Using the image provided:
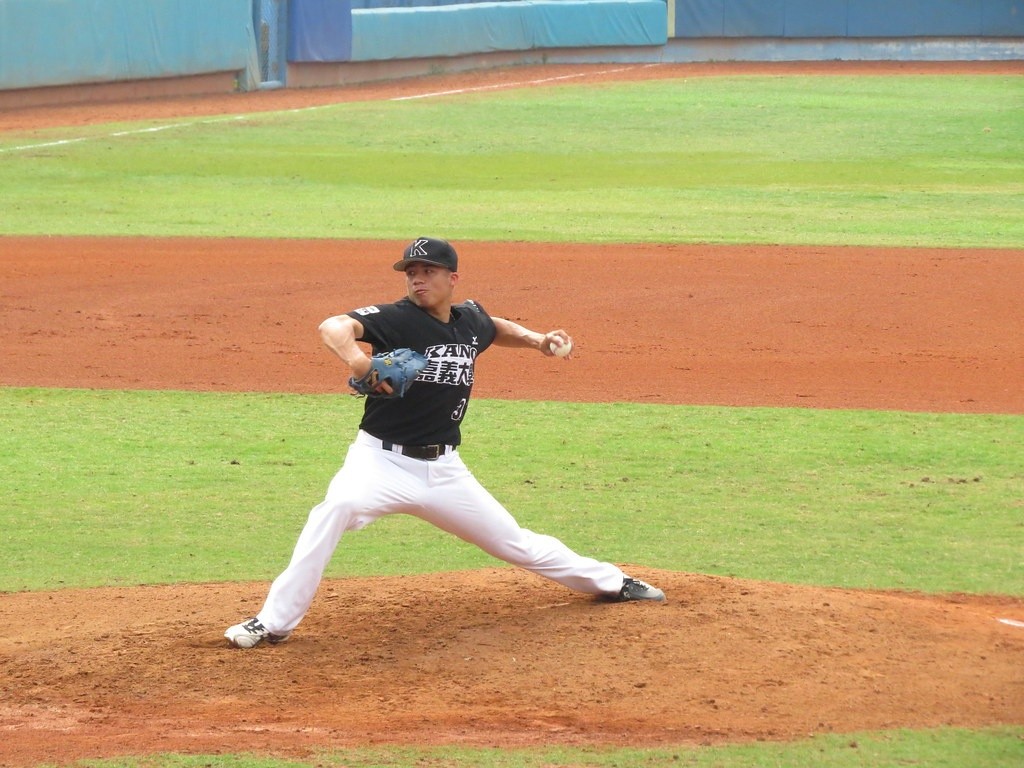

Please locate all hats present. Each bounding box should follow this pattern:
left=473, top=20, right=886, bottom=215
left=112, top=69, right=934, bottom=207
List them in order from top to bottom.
left=391, top=236, right=458, bottom=273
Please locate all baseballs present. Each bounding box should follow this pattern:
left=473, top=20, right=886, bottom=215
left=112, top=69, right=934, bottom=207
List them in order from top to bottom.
left=550, top=335, right=572, bottom=357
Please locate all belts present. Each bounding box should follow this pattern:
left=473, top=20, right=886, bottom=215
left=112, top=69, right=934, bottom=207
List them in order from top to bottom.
left=381, top=440, right=457, bottom=461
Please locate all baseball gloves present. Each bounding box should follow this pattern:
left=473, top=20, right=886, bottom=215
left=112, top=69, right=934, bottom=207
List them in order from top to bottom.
left=348, top=349, right=429, bottom=400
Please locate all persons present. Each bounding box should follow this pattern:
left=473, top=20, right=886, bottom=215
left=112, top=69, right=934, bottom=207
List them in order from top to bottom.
left=220, top=235, right=667, bottom=651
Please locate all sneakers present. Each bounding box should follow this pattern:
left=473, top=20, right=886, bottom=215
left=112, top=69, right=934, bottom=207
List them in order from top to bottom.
left=616, top=574, right=667, bottom=602
left=224, top=617, right=290, bottom=649
